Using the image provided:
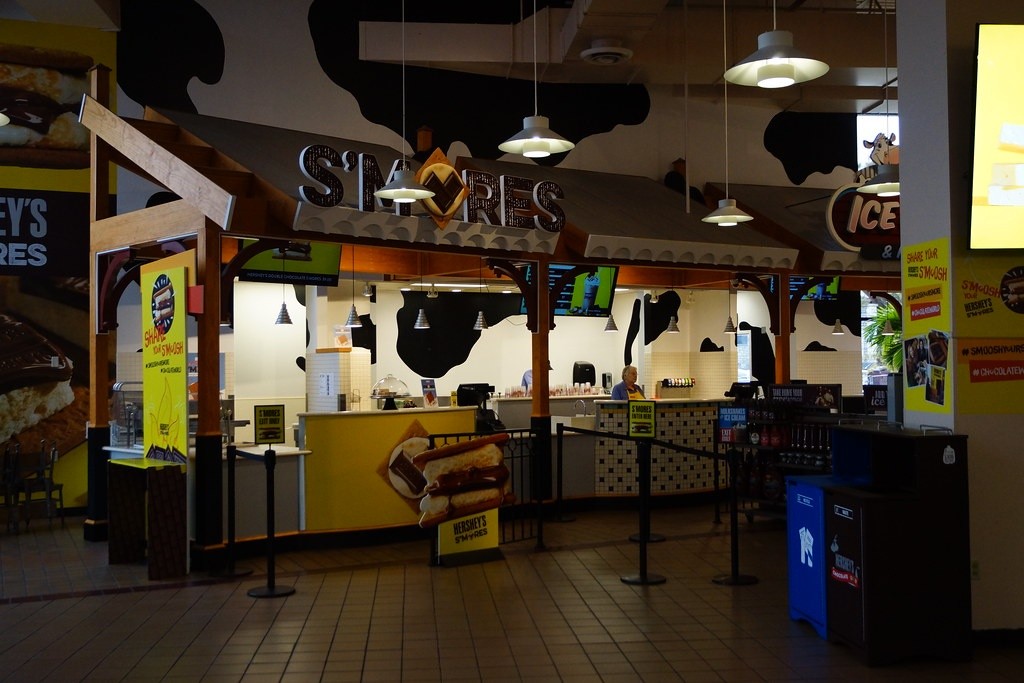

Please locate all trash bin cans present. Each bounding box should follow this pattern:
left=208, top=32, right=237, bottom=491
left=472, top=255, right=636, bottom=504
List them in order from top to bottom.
left=784, top=474, right=873, bottom=641
left=823, top=484, right=922, bottom=666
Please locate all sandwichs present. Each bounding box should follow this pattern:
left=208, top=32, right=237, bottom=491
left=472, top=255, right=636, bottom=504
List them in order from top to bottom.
left=0, top=41, right=95, bottom=170
left=0, top=309, right=75, bottom=444
left=412, top=433, right=517, bottom=528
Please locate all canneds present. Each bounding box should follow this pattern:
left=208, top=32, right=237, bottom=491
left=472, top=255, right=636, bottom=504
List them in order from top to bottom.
left=668, top=377, right=695, bottom=386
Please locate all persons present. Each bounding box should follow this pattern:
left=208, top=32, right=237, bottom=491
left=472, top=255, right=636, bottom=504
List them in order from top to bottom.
left=906, top=338, right=928, bottom=386
left=610, top=365, right=646, bottom=400
left=522, top=370, right=532, bottom=389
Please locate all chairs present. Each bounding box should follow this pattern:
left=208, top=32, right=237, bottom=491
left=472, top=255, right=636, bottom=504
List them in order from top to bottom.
left=0, top=438, right=65, bottom=535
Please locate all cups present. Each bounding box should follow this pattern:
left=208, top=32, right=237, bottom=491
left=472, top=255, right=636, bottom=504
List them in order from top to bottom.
left=583, top=275, right=600, bottom=311
left=505, top=382, right=600, bottom=399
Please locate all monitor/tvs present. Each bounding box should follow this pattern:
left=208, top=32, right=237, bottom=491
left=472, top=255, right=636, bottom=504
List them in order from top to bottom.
left=967, top=22, right=1024, bottom=255
left=236, top=239, right=342, bottom=289
left=520, top=264, right=619, bottom=318
left=771, top=275, right=841, bottom=301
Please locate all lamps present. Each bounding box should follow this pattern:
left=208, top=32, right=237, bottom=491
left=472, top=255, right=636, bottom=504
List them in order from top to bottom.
left=372, top=0, right=435, bottom=203
left=498, top=0, right=575, bottom=158
left=700, top=0, right=754, bottom=227
left=724, top=0, right=829, bottom=88
left=857, top=0, right=900, bottom=197
left=344, top=245, right=363, bottom=328
left=275, top=252, right=293, bottom=324
left=414, top=252, right=429, bottom=329
left=473, top=257, right=488, bottom=330
left=605, top=267, right=618, bottom=332
left=722, top=272, right=736, bottom=335
left=361, top=281, right=373, bottom=297
left=426, top=283, right=438, bottom=298
left=648, top=288, right=659, bottom=304
left=881, top=291, right=895, bottom=336
left=666, top=316, right=680, bottom=333
left=832, top=319, right=845, bottom=336
